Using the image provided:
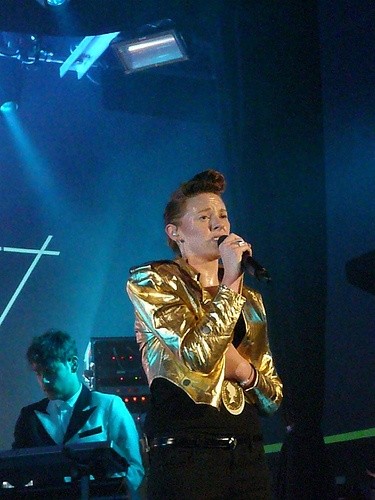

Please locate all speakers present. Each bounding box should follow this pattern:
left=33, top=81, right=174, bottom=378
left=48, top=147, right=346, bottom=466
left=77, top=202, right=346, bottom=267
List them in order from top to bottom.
left=83, top=337, right=149, bottom=414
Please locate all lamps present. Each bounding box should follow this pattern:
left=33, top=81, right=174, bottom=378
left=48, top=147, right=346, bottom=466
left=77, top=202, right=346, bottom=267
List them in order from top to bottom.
left=110, top=16, right=190, bottom=76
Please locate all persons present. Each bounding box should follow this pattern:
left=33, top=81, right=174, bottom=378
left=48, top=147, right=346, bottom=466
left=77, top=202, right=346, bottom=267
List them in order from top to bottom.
left=125, top=170, right=283, bottom=500
left=11, top=330, right=146, bottom=500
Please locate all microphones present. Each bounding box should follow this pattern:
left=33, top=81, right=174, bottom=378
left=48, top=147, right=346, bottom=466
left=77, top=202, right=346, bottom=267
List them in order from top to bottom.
left=217, top=235, right=273, bottom=284
